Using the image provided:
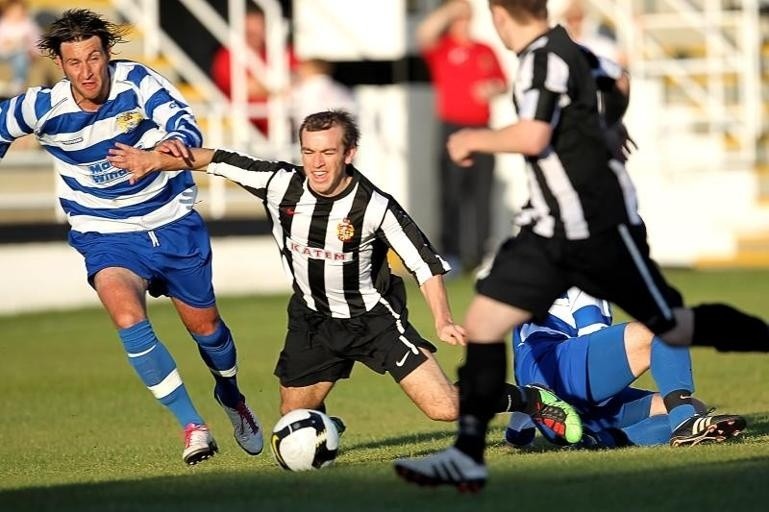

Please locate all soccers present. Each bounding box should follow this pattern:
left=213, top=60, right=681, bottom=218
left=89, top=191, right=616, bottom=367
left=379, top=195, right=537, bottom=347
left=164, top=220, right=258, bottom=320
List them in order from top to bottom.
left=270, top=409, right=340, bottom=472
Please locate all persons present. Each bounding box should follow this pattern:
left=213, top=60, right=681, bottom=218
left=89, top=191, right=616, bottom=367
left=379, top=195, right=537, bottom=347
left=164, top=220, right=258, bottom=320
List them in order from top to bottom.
left=388, top=0, right=768, bottom=495
left=556, top=0, right=629, bottom=118
left=0, top=1, right=43, bottom=92
left=415, top=1, right=508, bottom=274
left=205, top=5, right=324, bottom=142
left=0, top=6, right=265, bottom=467
left=104, top=109, right=585, bottom=451
left=505, top=283, right=747, bottom=450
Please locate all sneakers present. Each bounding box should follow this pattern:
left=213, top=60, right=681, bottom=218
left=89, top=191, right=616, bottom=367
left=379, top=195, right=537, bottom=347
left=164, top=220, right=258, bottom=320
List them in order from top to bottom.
left=526, top=383, right=584, bottom=444
left=214, top=385, right=263, bottom=455
left=669, top=408, right=747, bottom=448
left=182, top=422, right=218, bottom=466
left=573, top=426, right=604, bottom=451
left=393, top=447, right=488, bottom=494
